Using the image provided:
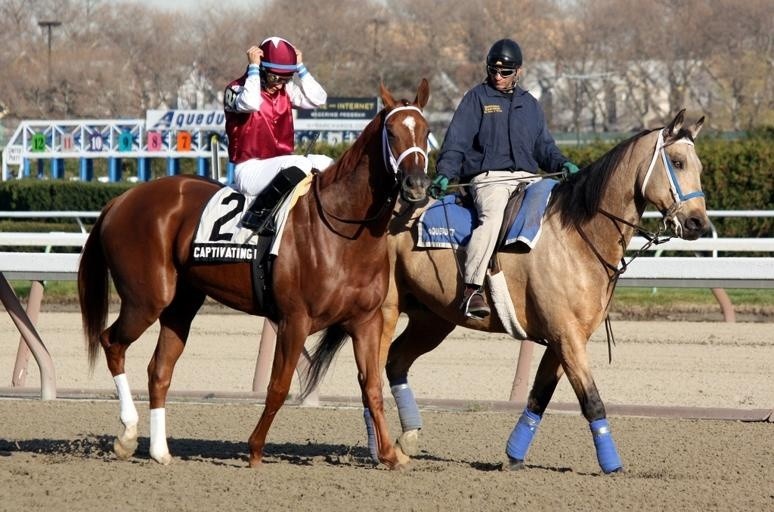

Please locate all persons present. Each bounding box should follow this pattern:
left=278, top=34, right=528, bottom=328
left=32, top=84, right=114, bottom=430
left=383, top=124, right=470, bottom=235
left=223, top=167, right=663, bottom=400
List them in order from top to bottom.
left=224, top=37, right=335, bottom=195
left=429, top=38, right=580, bottom=318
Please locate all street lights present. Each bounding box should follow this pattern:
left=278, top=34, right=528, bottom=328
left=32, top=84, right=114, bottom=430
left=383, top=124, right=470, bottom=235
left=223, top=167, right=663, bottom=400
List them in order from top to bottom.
left=361, top=16, right=393, bottom=102
left=36, top=19, right=66, bottom=119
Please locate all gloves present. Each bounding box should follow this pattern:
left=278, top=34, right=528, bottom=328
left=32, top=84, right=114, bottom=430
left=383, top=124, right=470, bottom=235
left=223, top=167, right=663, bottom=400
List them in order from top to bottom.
left=560, top=160, right=580, bottom=180
left=424, top=171, right=452, bottom=200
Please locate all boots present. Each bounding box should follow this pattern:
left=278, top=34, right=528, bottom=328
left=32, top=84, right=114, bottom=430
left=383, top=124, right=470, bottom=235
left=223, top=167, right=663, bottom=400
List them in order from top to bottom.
left=238, top=164, right=309, bottom=238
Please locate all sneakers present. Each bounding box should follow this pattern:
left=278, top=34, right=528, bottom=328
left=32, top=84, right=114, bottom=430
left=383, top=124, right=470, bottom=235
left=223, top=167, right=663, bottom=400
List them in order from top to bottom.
left=459, top=287, right=491, bottom=316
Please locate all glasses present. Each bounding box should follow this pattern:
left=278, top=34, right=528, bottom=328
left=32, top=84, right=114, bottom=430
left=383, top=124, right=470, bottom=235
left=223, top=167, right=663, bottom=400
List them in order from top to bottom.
left=487, top=67, right=516, bottom=78
left=268, top=74, right=292, bottom=84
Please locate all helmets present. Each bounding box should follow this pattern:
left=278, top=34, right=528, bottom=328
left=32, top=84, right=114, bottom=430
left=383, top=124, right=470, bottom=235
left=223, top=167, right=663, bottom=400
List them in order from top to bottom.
left=257, top=37, right=298, bottom=72
left=486, top=38, right=521, bottom=69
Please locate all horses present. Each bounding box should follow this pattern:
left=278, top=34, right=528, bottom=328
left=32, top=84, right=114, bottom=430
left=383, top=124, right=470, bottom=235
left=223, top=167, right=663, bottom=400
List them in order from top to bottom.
left=75, top=76, right=431, bottom=471
left=296, top=109, right=709, bottom=476
